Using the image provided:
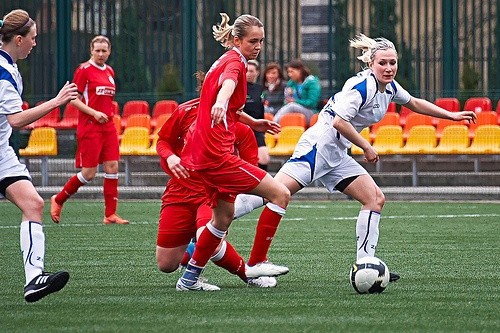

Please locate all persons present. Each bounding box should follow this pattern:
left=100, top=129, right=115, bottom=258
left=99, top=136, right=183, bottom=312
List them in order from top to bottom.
left=0, top=9, right=79, bottom=302
left=176, top=13, right=290, bottom=291
left=231, top=33, right=477, bottom=280
left=51, top=36, right=129, bottom=225
left=242, top=59, right=269, bottom=169
left=269, top=60, right=321, bottom=124
left=260, top=63, right=285, bottom=114
left=154, top=71, right=278, bottom=287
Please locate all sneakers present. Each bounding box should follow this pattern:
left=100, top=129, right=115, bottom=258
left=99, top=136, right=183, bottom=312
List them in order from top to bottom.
left=51, top=194, right=62, bottom=223
left=104, top=214, right=129, bottom=225
left=245, top=260, right=289, bottom=279
left=24, top=270, right=69, bottom=302
left=248, top=275, right=277, bottom=288
left=176, top=278, right=220, bottom=292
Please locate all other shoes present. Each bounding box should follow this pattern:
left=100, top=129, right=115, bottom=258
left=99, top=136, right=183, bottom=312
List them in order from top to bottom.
left=186, top=241, right=195, bottom=258
left=390, top=273, right=400, bottom=280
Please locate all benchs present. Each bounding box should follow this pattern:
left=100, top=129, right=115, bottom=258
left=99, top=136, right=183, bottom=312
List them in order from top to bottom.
left=269, top=97, right=500, bottom=186
left=20, top=101, right=178, bottom=187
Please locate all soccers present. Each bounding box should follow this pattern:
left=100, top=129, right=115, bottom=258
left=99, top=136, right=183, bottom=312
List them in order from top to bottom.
left=349, top=256, right=390, bottom=294
